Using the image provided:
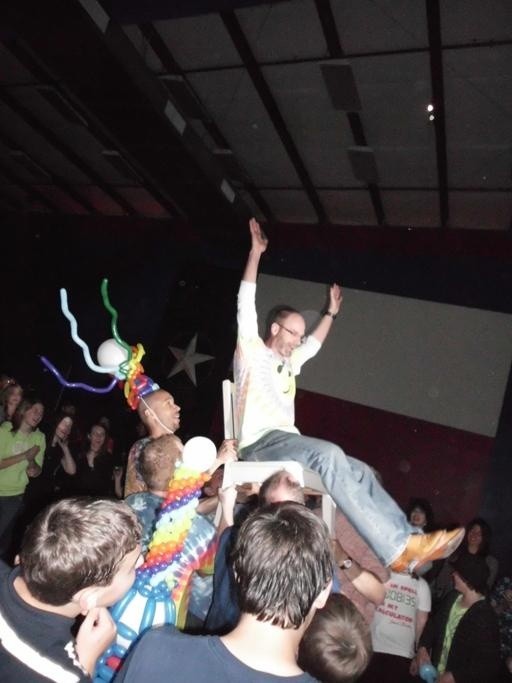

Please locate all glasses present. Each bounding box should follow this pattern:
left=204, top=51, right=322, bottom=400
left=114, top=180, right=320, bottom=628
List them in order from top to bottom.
left=280, top=323, right=307, bottom=343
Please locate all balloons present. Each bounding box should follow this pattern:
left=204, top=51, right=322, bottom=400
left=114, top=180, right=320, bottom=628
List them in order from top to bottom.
left=40, top=276, right=159, bottom=414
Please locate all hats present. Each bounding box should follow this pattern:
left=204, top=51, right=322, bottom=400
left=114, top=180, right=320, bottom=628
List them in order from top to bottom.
left=449, top=560, right=489, bottom=587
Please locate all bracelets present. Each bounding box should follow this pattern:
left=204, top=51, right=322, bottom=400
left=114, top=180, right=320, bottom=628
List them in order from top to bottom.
left=320, top=309, right=337, bottom=321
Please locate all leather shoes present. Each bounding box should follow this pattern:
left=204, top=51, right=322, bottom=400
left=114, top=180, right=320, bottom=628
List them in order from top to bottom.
left=413, top=526, right=466, bottom=577
left=390, top=528, right=445, bottom=572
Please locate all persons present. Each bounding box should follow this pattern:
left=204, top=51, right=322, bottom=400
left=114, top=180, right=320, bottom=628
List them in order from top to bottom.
left=233, top=217, right=468, bottom=574
left=1, top=376, right=512, bottom=683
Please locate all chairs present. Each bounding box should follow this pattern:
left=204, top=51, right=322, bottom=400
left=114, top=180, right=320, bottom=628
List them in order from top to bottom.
left=211, top=380, right=338, bottom=533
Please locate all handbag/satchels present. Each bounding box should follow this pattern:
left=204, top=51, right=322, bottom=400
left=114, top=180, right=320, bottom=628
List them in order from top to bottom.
left=39, top=469, right=76, bottom=501
